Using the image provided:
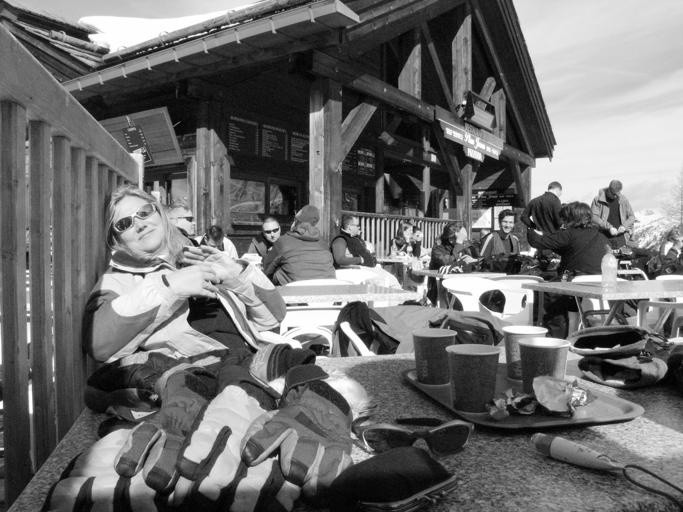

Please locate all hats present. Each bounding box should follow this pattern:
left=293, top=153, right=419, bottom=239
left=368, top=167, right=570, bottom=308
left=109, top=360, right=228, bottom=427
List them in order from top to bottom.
left=294, top=203, right=321, bottom=225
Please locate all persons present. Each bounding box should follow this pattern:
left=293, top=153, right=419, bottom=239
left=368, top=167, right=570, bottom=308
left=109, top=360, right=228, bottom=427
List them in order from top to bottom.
left=84, top=184, right=317, bottom=390
left=160, top=198, right=196, bottom=240
left=260, top=202, right=335, bottom=286
left=327, top=213, right=377, bottom=269
left=248, top=217, right=281, bottom=256
left=192, top=226, right=239, bottom=258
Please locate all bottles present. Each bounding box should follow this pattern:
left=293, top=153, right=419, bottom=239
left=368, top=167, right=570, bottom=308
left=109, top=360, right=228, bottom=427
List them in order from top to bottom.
left=599, top=247, right=619, bottom=287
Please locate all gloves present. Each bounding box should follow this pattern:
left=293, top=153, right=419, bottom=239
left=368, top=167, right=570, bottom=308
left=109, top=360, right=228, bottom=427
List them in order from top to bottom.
left=39, top=343, right=356, bottom=512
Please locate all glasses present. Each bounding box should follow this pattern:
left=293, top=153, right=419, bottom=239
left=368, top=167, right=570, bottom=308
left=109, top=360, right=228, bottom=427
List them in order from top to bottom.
left=109, top=201, right=159, bottom=233
left=345, top=223, right=360, bottom=228
left=263, top=227, right=280, bottom=234
left=350, top=406, right=473, bottom=455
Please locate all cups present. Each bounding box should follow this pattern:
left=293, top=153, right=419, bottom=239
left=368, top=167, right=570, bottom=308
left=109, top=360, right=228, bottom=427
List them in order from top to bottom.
left=502, top=325, right=549, bottom=382
left=412, top=328, right=457, bottom=388
left=519, top=336, right=571, bottom=398
left=444, top=343, right=499, bottom=415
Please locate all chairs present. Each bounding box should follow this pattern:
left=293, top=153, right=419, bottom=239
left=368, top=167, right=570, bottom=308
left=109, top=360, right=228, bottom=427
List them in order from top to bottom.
left=334, top=262, right=404, bottom=293
left=572, top=268, right=683, bottom=282
left=278, top=278, right=368, bottom=337
left=330, top=300, right=504, bottom=358
left=566, top=300, right=683, bottom=350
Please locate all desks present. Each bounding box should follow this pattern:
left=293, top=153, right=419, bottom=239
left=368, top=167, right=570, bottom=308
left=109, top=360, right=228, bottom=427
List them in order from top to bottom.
left=442, top=275, right=545, bottom=327
